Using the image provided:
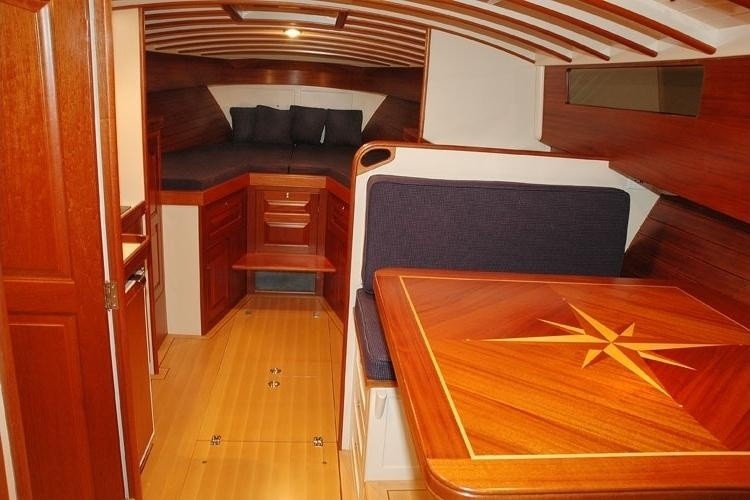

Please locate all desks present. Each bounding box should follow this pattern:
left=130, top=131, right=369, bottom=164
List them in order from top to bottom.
left=372, top=266, right=750, bottom=500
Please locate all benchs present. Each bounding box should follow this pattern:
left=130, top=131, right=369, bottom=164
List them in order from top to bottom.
left=348, top=174, right=630, bottom=482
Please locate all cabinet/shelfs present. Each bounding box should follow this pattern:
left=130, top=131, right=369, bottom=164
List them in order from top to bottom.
left=88, top=0, right=155, bottom=476
left=162, top=174, right=247, bottom=336
left=247, top=174, right=323, bottom=297
left=323, top=177, right=355, bottom=324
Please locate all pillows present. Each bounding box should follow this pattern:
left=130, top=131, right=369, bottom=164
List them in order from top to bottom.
left=256, top=104, right=296, bottom=148
left=290, top=104, right=326, bottom=149
left=230, top=106, right=255, bottom=147
left=326, top=109, right=363, bottom=148
